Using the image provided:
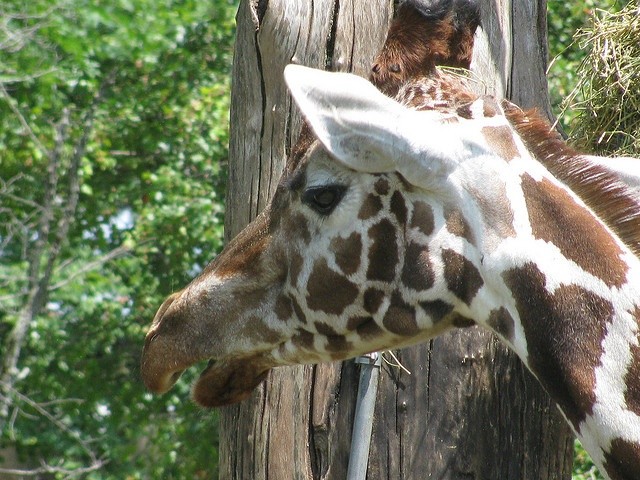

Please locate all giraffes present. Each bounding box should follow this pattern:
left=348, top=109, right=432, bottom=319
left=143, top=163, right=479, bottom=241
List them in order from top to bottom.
left=141, top=0, right=640, bottom=480
